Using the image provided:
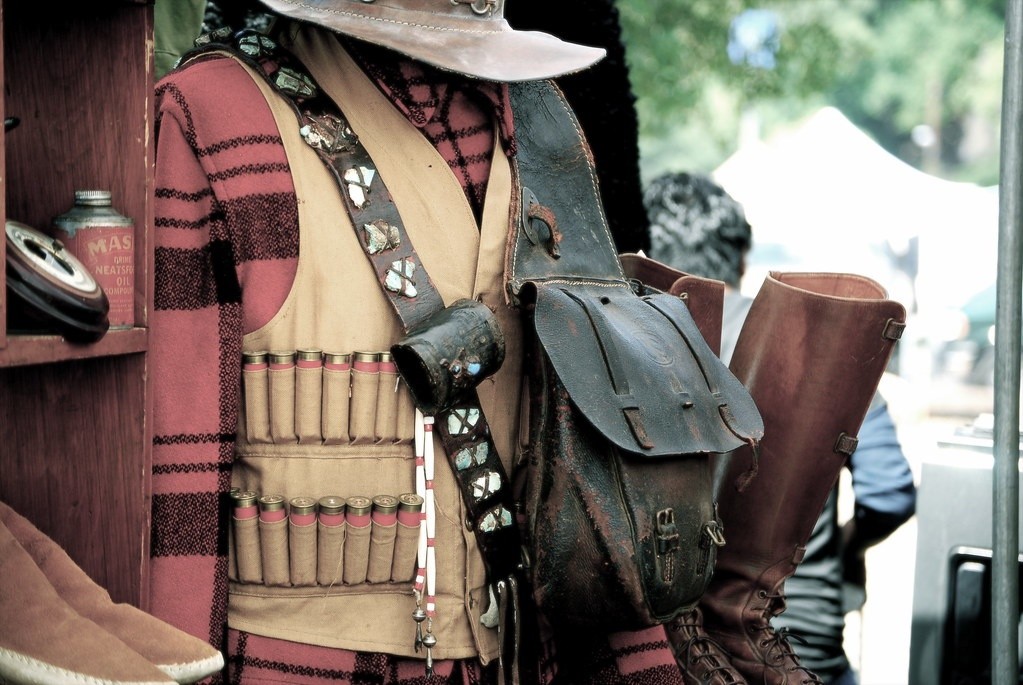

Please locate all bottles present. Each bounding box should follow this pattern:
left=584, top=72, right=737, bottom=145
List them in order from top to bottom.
left=54, top=190, right=135, bottom=329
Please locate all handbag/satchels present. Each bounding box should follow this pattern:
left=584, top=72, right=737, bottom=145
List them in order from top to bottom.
left=497, top=81, right=765, bottom=634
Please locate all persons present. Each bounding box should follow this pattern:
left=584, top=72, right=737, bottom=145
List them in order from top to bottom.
left=150, top=1, right=681, bottom=685
left=642, top=169, right=917, bottom=685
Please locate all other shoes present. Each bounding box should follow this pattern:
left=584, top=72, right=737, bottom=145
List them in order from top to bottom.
left=0, top=502, right=225, bottom=685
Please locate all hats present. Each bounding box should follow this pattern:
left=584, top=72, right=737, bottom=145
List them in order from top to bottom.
left=260, top=0, right=605, bottom=84
left=644, top=170, right=753, bottom=292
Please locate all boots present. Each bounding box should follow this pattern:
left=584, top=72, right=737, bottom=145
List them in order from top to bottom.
left=592, top=253, right=748, bottom=685
left=705, top=271, right=905, bottom=685
